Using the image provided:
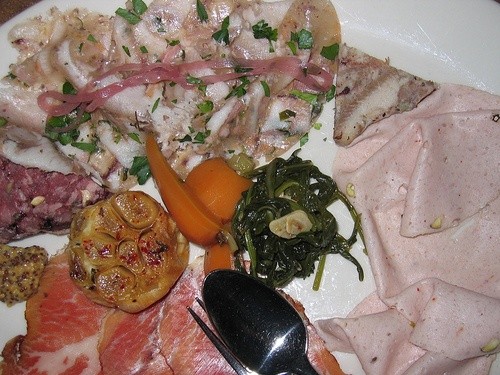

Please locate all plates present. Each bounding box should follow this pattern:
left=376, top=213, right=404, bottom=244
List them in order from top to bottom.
left=0, top=0, right=499, bottom=375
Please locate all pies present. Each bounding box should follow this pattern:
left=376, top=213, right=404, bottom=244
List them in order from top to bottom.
left=316, top=84, right=500, bottom=374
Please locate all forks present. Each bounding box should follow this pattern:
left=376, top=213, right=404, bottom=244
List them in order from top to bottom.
left=188, top=298, right=252, bottom=375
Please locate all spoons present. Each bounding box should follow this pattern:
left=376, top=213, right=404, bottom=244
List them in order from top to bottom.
left=202, top=269, right=321, bottom=375
